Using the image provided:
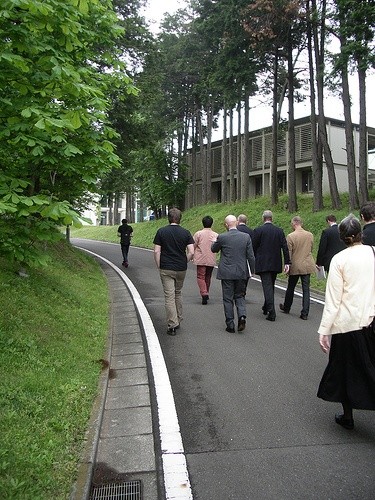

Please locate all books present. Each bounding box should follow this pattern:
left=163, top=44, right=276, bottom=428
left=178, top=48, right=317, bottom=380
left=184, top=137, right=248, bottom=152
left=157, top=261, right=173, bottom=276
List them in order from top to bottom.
left=317, top=266, right=326, bottom=279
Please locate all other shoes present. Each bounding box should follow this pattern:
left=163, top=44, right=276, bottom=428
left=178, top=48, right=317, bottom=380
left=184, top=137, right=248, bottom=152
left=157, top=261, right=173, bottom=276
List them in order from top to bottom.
left=122, top=261, right=128, bottom=267
left=202, top=295, right=209, bottom=304
left=280, top=303, right=289, bottom=313
left=262, top=306, right=267, bottom=315
left=300, top=315, right=307, bottom=319
left=237, top=317, right=246, bottom=331
left=266, top=317, right=275, bottom=321
left=167, top=322, right=180, bottom=336
left=226, top=328, right=235, bottom=333
left=335, top=414, right=354, bottom=429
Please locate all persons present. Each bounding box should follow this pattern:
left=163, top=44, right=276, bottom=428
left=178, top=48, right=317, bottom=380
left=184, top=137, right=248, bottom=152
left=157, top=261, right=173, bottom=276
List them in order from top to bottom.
left=316, top=206, right=375, bottom=430
left=153, top=208, right=195, bottom=335
left=251, top=210, right=316, bottom=321
left=211, top=214, right=256, bottom=333
left=191, top=216, right=220, bottom=305
left=117, top=219, right=134, bottom=267
